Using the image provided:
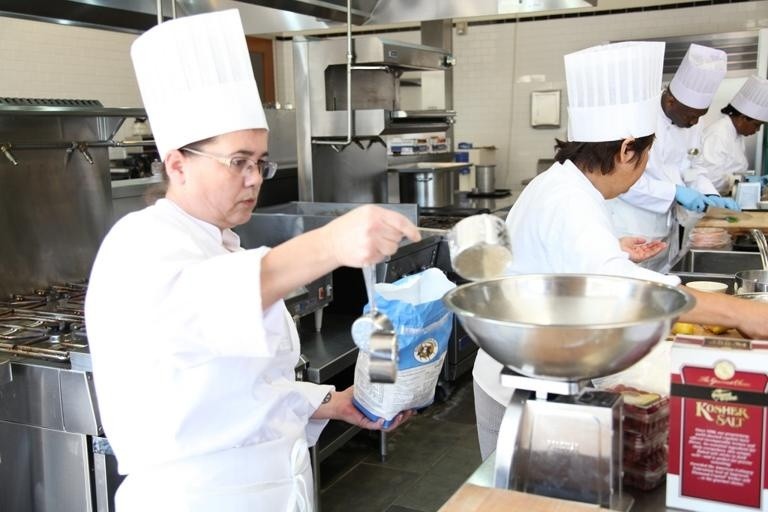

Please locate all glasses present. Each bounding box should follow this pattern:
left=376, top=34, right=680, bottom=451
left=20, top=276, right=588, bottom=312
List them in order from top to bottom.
left=180, top=145, right=278, bottom=182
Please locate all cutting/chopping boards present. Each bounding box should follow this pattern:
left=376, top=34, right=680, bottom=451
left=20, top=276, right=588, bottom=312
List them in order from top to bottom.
left=695, top=211, right=768, bottom=236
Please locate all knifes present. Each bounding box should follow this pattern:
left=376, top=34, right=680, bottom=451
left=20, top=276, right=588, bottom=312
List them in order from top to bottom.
left=676, top=200, right=752, bottom=220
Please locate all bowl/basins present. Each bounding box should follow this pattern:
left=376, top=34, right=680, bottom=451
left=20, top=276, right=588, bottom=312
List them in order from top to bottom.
left=445, top=273, right=696, bottom=381
left=686, top=281, right=729, bottom=294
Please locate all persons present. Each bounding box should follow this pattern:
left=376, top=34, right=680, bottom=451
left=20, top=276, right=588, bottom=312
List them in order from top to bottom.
left=81, top=6, right=422, bottom=511
left=470, top=38, right=768, bottom=463
left=608, top=43, right=743, bottom=270
left=701, top=76, right=768, bottom=189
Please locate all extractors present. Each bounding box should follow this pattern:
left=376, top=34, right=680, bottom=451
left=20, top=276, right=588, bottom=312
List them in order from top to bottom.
left=0, top=2, right=173, bottom=116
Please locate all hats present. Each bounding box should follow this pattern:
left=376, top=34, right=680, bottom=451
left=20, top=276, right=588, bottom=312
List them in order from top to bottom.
left=129, top=7, right=269, bottom=161
left=565, top=41, right=665, bottom=142
left=669, top=43, right=728, bottom=110
left=731, top=74, right=768, bottom=124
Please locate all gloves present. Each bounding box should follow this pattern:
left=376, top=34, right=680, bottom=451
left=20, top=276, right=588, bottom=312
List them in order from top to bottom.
left=745, top=176, right=768, bottom=188
left=676, top=186, right=716, bottom=214
left=708, top=195, right=742, bottom=214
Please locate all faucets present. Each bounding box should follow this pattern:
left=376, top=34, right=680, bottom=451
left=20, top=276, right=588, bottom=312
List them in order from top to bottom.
left=748, top=229, right=768, bottom=270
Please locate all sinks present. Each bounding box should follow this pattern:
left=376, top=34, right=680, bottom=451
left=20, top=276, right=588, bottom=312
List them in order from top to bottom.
left=668, top=246, right=766, bottom=275
left=675, top=279, right=742, bottom=299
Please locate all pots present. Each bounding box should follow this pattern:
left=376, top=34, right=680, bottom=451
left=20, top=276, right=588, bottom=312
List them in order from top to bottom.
left=413, top=171, right=454, bottom=208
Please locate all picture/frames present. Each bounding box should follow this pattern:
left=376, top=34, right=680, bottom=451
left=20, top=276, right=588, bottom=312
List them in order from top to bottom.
left=530, top=90, right=562, bottom=128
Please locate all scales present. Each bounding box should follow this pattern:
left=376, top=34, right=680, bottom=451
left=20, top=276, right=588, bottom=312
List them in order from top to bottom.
left=442, top=275, right=697, bottom=508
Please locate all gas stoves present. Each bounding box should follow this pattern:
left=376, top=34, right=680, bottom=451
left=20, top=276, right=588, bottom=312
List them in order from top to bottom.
left=0, top=278, right=93, bottom=372
